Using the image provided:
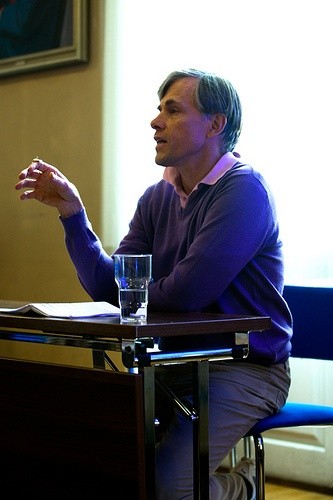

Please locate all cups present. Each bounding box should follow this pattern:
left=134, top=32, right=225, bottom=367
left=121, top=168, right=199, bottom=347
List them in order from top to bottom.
left=113, top=253, right=152, bottom=325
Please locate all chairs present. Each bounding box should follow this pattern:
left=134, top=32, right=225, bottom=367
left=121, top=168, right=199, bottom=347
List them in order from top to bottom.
left=244, top=286, right=333, bottom=500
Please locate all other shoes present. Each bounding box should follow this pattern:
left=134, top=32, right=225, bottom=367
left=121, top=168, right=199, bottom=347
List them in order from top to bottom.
left=234, top=457, right=256, bottom=500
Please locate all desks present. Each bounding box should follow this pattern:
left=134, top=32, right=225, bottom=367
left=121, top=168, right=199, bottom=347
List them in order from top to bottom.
left=0, top=299, right=270, bottom=500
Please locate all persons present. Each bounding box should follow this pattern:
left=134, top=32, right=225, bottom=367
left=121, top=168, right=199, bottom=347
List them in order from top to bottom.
left=15, top=68, right=293, bottom=500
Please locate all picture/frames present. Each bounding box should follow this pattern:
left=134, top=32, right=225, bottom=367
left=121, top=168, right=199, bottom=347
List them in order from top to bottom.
left=0, top=0, right=90, bottom=79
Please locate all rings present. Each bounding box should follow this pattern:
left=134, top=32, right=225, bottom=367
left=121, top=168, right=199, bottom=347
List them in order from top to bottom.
left=32, top=156, right=42, bottom=163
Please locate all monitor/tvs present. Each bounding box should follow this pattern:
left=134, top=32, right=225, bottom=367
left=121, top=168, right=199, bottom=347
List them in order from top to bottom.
left=0, top=0, right=89, bottom=76
